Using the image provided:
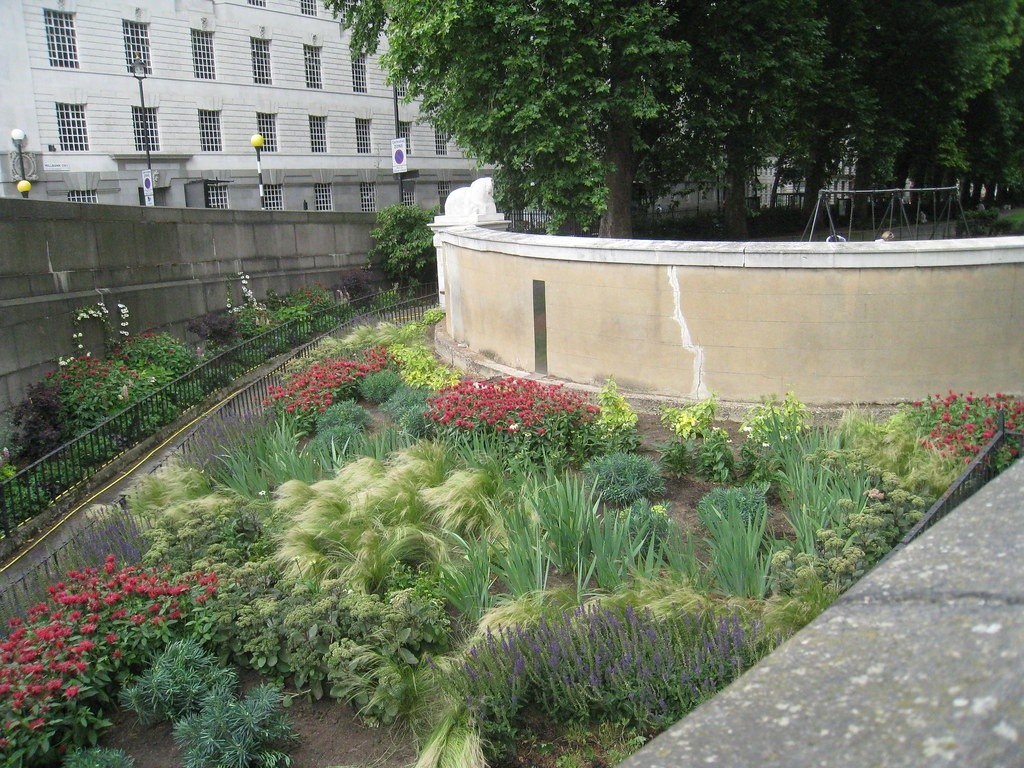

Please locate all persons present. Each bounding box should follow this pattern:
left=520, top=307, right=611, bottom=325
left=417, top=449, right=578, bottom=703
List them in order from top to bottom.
left=976, top=200, right=985, bottom=210
left=656, top=204, right=662, bottom=217
left=875, top=231, right=894, bottom=241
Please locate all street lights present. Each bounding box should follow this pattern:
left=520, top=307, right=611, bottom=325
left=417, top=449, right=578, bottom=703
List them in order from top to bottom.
left=130, top=56, right=152, bottom=171
left=11, top=128, right=26, bottom=181
left=250, top=135, right=267, bottom=210
